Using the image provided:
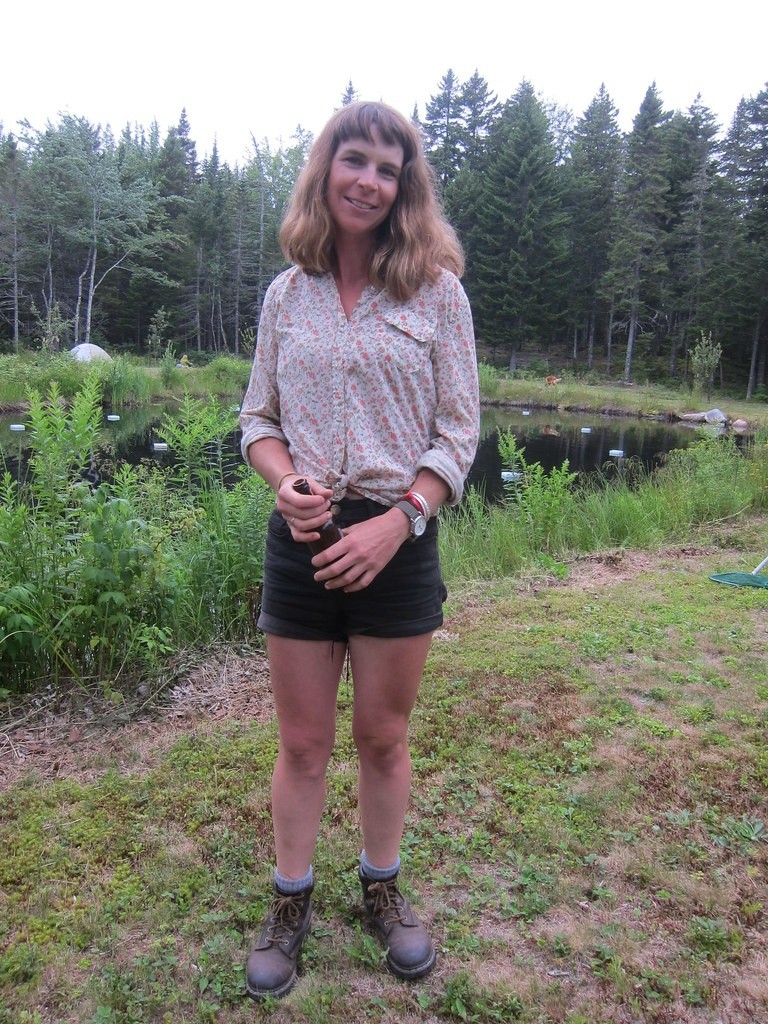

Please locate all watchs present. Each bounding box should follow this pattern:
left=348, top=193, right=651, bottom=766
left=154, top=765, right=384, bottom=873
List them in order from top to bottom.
left=394, top=500, right=426, bottom=539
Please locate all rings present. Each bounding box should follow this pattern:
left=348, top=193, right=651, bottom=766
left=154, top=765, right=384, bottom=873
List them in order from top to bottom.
left=289, top=517, right=295, bottom=525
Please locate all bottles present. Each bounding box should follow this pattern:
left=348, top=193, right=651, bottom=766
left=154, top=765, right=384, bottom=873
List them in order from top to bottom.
left=292, top=478, right=346, bottom=591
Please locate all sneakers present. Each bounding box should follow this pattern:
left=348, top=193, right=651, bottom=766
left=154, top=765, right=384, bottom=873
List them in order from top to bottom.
left=358, top=867, right=436, bottom=978
left=245, top=883, right=313, bottom=1001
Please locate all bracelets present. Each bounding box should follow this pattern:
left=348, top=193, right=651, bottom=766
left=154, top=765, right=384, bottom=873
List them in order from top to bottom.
left=278, top=472, right=300, bottom=490
left=398, top=492, right=430, bottom=522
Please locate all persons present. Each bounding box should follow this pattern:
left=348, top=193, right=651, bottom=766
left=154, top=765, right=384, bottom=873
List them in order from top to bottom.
left=237, top=100, right=479, bottom=1003
